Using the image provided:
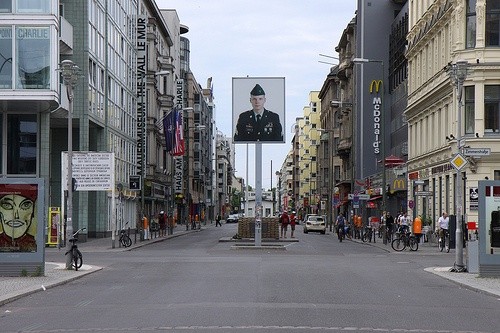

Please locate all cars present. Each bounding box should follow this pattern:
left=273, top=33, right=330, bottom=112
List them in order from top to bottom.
left=226, top=213, right=245, bottom=223
left=268, top=214, right=299, bottom=224
left=304, top=214, right=326, bottom=235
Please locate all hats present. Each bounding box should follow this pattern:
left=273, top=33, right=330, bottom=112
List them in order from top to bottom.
left=250, top=84, right=265, bottom=96
left=284, top=210, right=287, bottom=212
left=292, top=211, right=296, bottom=213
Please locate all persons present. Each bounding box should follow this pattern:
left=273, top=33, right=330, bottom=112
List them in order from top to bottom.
left=234, top=83, right=283, bottom=141
left=142, top=211, right=168, bottom=240
left=280, top=211, right=289, bottom=238
left=289, top=211, right=297, bottom=237
left=335, top=211, right=450, bottom=250
left=216, top=213, right=222, bottom=227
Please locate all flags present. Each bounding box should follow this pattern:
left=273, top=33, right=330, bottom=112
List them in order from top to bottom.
left=164, top=106, right=184, bottom=156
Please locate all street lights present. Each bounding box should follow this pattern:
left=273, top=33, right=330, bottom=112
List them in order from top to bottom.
left=352, top=58, right=388, bottom=243
left=55, top=60, right=82, bottom=270
left=449, top=61, right=469, bottom=273
left=140, top=70, right=169, bottom=242
left=169, top=108, right=194, bottom=234
left=185, top=125, right=206, bottom=231
left=317, top=129, right=334, bottom=231
left=210, top=158, right=222, bottom=223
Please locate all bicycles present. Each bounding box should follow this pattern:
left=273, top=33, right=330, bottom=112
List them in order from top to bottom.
left=337, top=222, right=372, bottom=242
left=118, top=223, right=132, bottom=247
left=379, top=223, right=418, bottom=252
left=65, top=227, right=87, bottom=271
left=439, top=228, right=450, bottom=253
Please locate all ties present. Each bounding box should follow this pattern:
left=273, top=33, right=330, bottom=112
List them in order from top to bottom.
left=256, top=114, right=261, bottom=122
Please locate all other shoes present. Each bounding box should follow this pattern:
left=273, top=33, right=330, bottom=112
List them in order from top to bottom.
left=291, top=236, right=295, bottom=238
left=280, top=237, right=282, bottom=238
left=284, top=237, right=287, bottom=238
